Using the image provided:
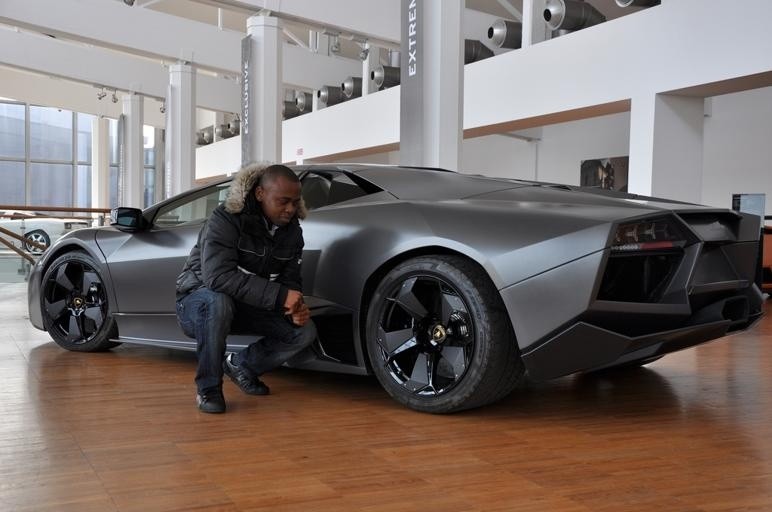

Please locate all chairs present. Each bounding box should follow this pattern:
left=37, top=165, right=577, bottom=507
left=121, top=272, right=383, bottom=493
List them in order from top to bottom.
left=301, top=176, right=330, bottom=207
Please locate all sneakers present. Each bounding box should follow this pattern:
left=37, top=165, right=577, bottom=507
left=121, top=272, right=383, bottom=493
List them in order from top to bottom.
left=221, top=352, right=270, bottom=396
left=194, top=390, right=227, bottom=414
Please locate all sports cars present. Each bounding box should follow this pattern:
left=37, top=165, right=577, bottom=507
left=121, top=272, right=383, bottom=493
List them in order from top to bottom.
left=22, top=157, right=767, bottom=417
left=0, top=208, right=90, bottom=259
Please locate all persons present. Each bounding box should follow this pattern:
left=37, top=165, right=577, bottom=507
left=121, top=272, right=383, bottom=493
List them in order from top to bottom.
left=174, top=162, right=317, bottom=414
